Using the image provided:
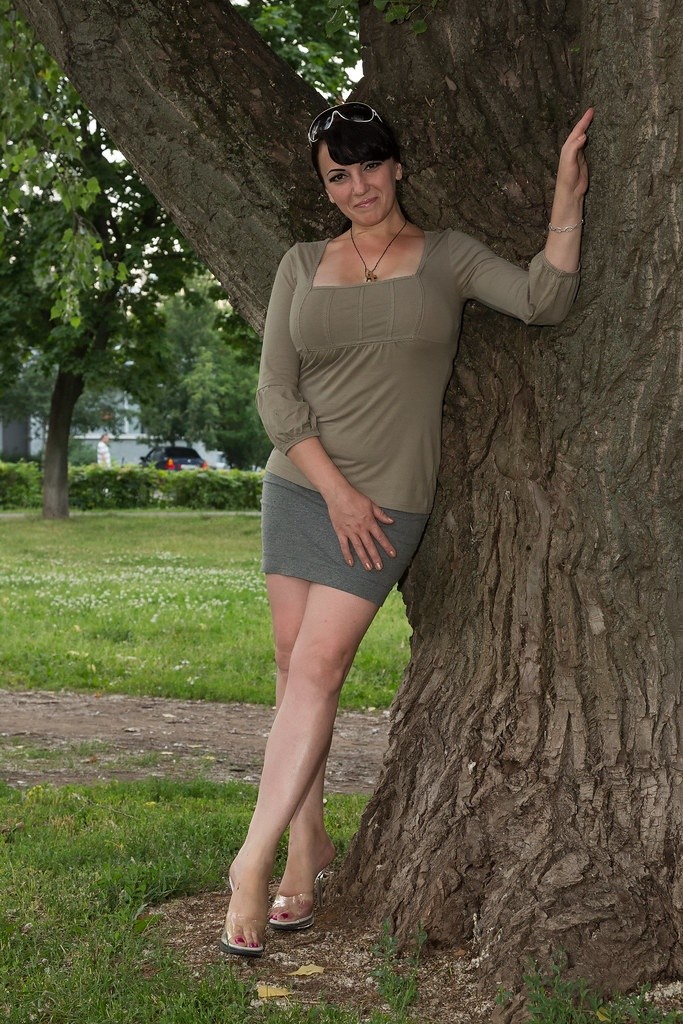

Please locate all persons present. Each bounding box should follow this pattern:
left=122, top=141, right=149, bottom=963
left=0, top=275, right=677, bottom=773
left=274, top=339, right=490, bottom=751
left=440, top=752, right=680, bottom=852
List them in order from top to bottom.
left=218, top=102, right=597, bottom=958
left=96, top=434, right=113, bottom=467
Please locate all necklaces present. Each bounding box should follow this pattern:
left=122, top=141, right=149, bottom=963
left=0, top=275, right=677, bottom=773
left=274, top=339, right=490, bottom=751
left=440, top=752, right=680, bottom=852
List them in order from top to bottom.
left=349, top=220, right=409, bottom=282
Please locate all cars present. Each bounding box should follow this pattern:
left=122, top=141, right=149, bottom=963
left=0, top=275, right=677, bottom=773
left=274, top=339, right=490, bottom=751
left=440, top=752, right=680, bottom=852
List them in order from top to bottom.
left=139, top=445, right=206, bottom=471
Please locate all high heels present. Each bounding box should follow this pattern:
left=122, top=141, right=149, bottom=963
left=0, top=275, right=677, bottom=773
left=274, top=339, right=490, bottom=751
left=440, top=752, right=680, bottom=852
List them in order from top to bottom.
left=268, top=867, right=328, bottom=932
left=221, top=876, right=265, bottom=954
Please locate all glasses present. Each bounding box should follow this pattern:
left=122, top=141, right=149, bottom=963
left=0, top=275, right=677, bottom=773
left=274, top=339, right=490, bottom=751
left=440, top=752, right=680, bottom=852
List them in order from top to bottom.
left=307, top=102, right=381, bottom=150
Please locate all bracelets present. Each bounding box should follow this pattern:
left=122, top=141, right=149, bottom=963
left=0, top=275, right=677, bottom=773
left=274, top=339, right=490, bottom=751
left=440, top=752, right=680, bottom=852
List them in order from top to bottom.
left=549, top=219, right=584, bottom=233
